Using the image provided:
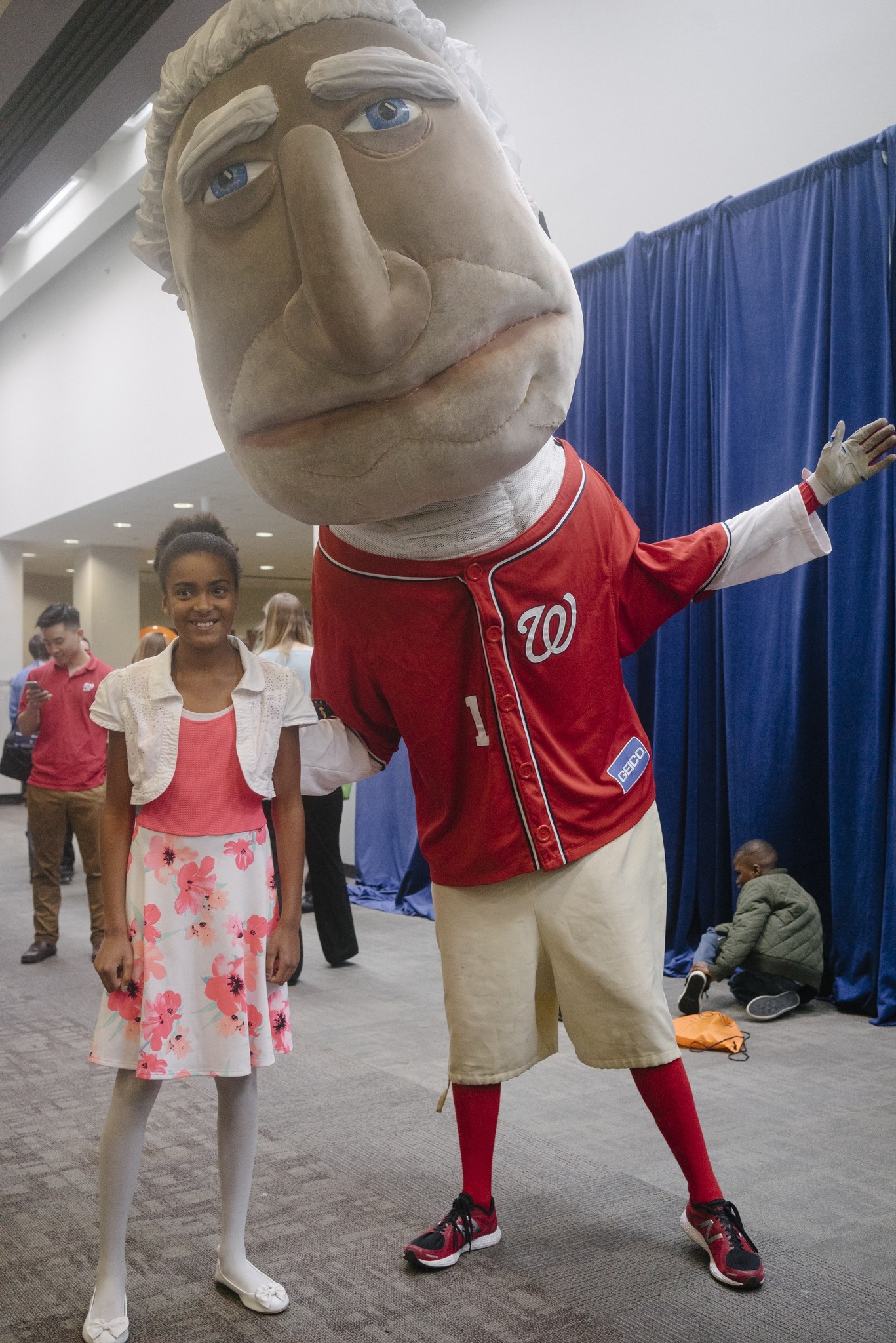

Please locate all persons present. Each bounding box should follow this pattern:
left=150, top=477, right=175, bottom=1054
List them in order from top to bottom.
left=126, top=3, right=895, bottom=1291
left=82, top=512, right=319, bottom=1343
left=251, top=592, right=358, bottom=985
left=9, top=604, right=181, bottom=964
left=678, top=840, right=822, bottom=1020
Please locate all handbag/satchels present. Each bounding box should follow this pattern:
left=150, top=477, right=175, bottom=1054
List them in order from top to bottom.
left=0, top=719, right=34, bottom=781
left=673, top=1011, right=751, bottom=1061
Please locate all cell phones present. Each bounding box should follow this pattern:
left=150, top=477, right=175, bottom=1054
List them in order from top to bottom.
left=25, top=681, right=44, bottom=699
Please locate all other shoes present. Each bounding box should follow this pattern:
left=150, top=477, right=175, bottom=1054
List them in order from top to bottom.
left=60, top=869, right=74, bottom=885
left=678, top=970, right=707, bottom=1015
left=745, top=991, right=801, bottom=1018
left=213, top=1244, right=290, bottom=1315
left=82, top=1281, right=129, bottom=1342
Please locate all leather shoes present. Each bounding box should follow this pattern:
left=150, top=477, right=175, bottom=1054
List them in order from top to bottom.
left=20, top=940, right=57, bottom=965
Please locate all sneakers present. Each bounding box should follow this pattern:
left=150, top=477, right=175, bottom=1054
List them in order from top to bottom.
left=400, top=1192, right=502, bottom=1269
left=680, top=1196, right=765, bottom=1292
